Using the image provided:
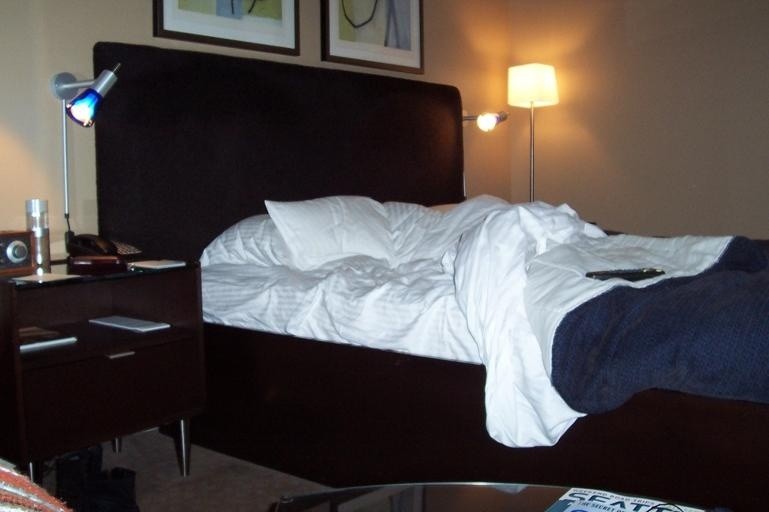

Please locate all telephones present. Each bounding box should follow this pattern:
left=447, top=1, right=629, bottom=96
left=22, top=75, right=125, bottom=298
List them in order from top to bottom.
left=68, top=234, right=143, bottom=259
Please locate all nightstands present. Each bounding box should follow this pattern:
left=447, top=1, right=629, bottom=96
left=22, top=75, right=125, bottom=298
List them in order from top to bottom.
left=1, top=253, right=202, bottom=483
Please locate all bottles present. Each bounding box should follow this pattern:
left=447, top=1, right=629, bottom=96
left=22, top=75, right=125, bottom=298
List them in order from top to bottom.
left=26, top=198, right=51, bottom=274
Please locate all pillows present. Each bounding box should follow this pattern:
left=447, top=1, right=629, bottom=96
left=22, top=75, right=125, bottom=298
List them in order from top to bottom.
left=199, top=194, right=396, bottom=272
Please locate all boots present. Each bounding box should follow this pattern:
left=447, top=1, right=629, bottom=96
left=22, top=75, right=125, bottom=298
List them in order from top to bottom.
left=55, top=444, right=103, bottom=509
left=82, top=467, right=141, bottom=512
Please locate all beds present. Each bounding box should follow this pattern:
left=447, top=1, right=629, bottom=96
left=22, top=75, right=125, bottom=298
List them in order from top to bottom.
left=192, top=202, right=769, bottom=509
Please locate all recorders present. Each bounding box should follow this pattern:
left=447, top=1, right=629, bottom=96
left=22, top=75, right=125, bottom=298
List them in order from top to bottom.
left=0, top=230, right=38, bottom=280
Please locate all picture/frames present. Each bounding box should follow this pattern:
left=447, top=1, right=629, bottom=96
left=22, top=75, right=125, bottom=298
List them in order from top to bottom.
left=153, top=1, right=424, bottom=76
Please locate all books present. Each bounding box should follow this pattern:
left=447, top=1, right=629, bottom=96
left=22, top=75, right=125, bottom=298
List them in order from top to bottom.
left=88, top=315, right=171, bottom=333
left=18, top=324, right=79, bottom=351
left=543, top=486, right=704, bottom=512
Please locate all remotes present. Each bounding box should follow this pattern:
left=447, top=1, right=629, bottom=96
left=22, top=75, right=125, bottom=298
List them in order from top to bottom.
left=585, top=266, right=666, bottom=282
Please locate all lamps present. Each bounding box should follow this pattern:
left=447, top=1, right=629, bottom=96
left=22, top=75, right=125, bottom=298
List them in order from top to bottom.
left=53, top=63, right=118, bottom=129
left=508, top=63, right=559, bottom=203
left=464, top=112, right=509, bottom=132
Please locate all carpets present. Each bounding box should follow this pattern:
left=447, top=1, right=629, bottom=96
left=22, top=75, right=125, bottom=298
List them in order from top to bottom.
left=38, top=430, right=338, bottom=508
